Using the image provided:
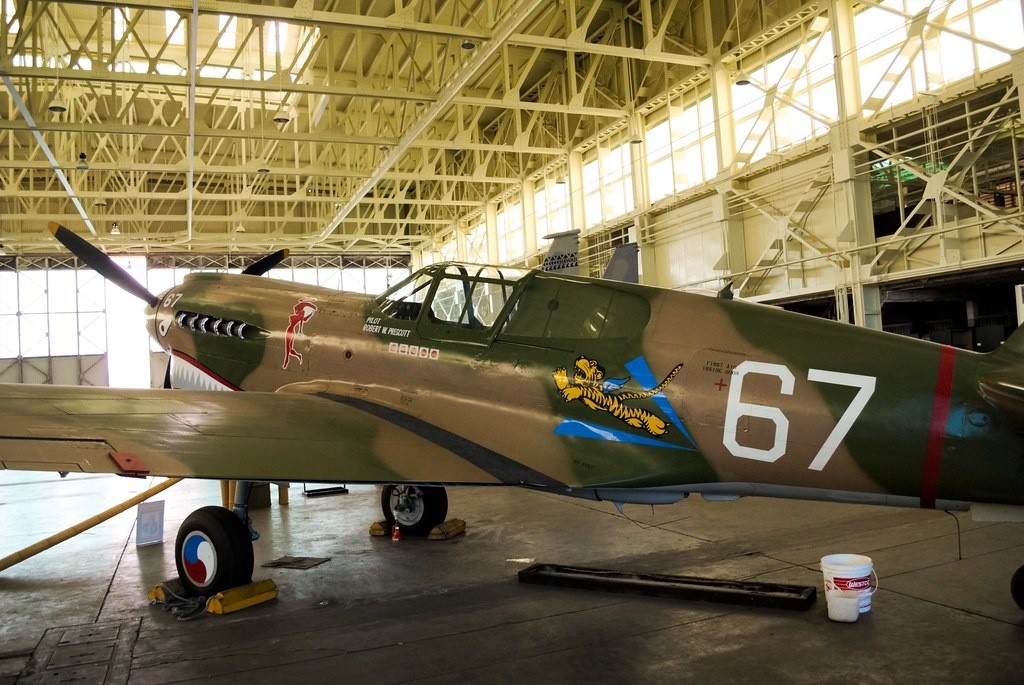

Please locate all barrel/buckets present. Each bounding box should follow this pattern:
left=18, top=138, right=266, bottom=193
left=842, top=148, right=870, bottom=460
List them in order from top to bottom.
left=820, top=554, right=878, bottom=614
left=825, top=590, right=859, bottom=622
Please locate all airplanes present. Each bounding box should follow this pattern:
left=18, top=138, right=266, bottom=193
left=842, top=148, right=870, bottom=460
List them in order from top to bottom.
left=0, top=218, right=1024, bottom=613
